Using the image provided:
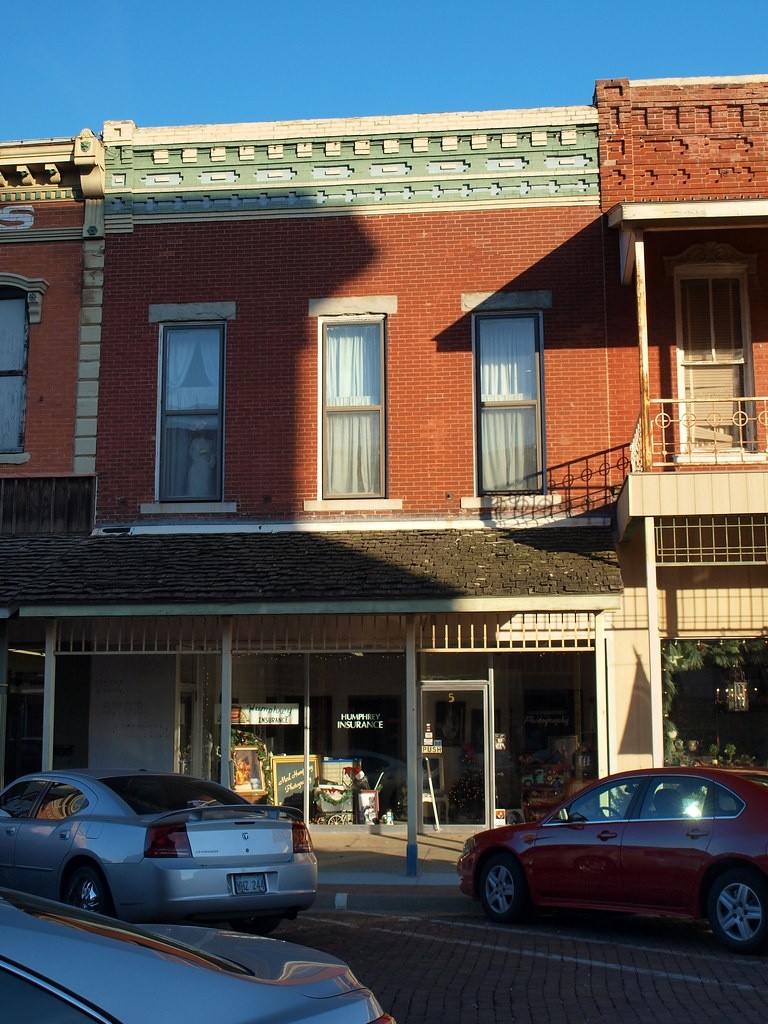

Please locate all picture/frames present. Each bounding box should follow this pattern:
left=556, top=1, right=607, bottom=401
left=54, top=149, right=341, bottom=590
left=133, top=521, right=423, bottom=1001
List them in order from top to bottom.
left=231, top=744, right=268, bottom=793
left=269, top=754, right=320, bottom=806
left=355, top=790, right=379, bottom=823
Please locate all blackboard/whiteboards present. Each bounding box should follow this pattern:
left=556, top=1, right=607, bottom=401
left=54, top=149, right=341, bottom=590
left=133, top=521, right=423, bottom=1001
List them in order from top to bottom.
left=272, top=758, right=318, bottom=811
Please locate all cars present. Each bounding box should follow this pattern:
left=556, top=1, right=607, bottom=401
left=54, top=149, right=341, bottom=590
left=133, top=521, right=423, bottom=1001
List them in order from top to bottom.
left=331, top=749, right=437, bottom=818
left=456, top=764, right=767, bottom=952
left=0, top=767, right=318, bottom=937
left=0, top=886, right=400, bottom=1023
